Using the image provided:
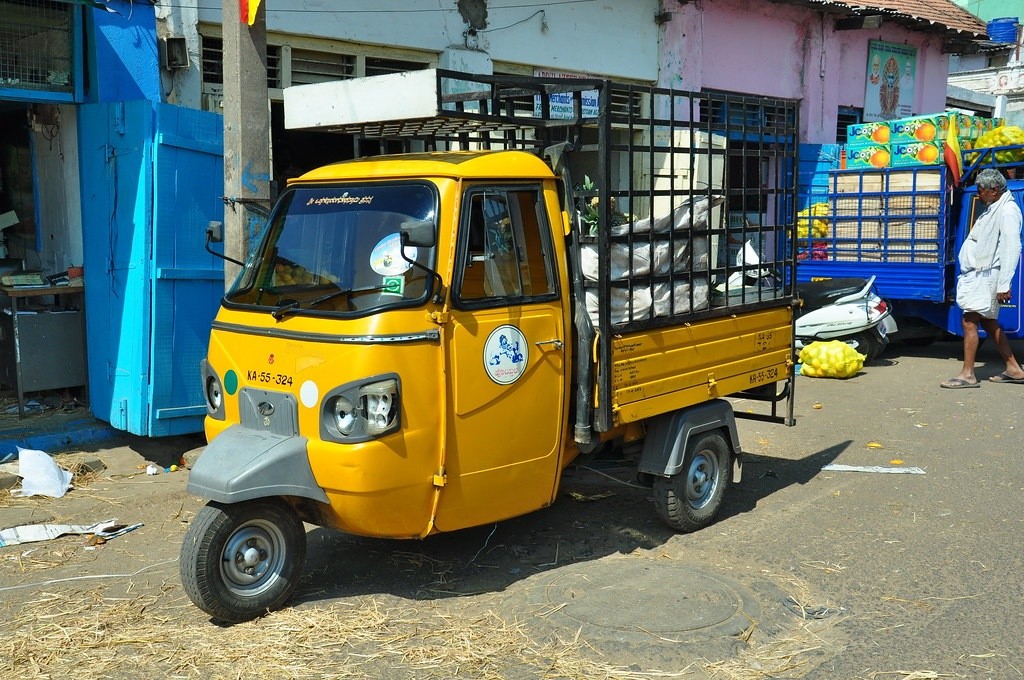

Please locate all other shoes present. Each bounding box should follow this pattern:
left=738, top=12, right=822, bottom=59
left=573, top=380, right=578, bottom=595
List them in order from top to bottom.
left=0, top=451, right=13, bottom=464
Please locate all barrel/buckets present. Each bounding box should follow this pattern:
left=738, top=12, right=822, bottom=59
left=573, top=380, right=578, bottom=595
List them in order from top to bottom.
left=986, top=17, right=1019, bottom=43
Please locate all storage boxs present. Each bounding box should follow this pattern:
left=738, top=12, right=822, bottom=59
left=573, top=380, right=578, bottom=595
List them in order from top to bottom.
left=827, top=113, right=1004, bottom=264
left=716, top=210, right=766, bottom=266
left=2, top=270, right=46, bottom=286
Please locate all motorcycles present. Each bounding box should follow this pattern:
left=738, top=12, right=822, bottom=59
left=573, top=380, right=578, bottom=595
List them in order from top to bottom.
left=175, top=78, right=805, bottom=626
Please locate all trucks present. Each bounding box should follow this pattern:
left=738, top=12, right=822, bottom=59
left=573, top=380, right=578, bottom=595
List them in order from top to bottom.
left=777, top=144, right=1024, bottom=343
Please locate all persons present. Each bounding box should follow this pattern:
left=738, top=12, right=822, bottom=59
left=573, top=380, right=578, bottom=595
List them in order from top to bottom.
left=940, top=168, right=1024, bottom=389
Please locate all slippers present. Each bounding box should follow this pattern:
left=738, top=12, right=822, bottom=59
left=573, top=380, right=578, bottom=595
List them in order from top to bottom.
left=991, top=373, right=1024, bottom=384
left=940, top=378, right=980, bottom=389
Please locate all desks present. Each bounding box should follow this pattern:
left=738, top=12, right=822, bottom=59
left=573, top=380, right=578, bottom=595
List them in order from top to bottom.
left=0, top=283, right=90, bottom=420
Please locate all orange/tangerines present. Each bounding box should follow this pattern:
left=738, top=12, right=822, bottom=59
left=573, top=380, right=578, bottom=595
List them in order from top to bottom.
left=871, top=124, right=939, bottom=167
left=969, top=126, right=1024, bottom=162
left=789, top=202, right=830, bottom=238
left=799, top=340, right=863, bottom=378
